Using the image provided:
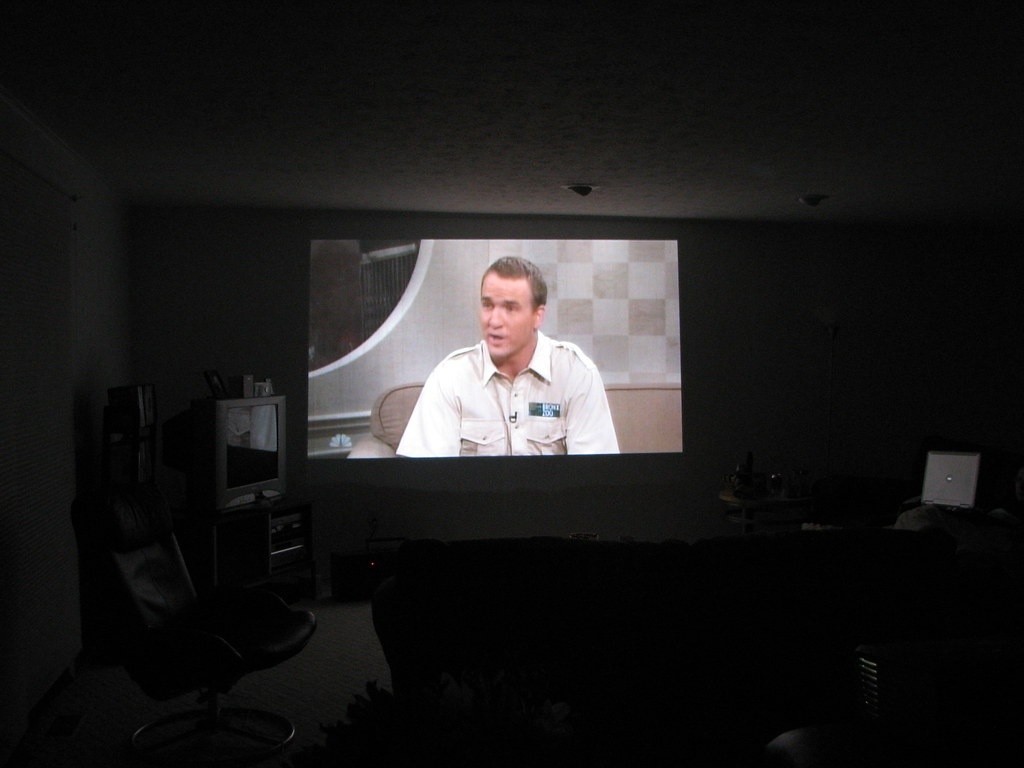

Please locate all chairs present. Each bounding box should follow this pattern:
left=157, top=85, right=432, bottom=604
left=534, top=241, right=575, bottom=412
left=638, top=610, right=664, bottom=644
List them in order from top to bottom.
left=104, top=497, right=318, bottom=767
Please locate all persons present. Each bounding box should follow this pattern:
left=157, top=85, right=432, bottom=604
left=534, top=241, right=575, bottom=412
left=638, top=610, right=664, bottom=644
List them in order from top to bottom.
left=396, top=257, right=621, bottom=457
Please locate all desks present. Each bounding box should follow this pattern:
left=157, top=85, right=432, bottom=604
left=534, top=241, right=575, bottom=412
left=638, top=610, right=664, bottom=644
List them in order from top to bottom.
left=718, top=489, right=817, bottom=534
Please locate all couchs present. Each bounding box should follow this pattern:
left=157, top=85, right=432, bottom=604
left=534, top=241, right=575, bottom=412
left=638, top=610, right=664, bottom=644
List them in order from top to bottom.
left=345, top=382, right=682, bottom=458
left=369, top=525, right=1024, bottom=768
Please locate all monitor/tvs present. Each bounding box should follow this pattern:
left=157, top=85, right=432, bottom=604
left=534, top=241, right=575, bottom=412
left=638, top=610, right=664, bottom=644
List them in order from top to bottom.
left=162, top=395, right=293, bottom=507
left=921, top=450, right=980, bottom=511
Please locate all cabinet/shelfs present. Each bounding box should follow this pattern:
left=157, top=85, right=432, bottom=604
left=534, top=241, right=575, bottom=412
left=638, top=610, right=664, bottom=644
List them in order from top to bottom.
left=100, top=385, right=155, bottom=494
left=175, top=500, right=318, bottom=591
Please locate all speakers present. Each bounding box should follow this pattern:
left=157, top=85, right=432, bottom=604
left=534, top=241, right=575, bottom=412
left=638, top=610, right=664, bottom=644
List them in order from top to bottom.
left=332, top=553, right=372, bottom=596
